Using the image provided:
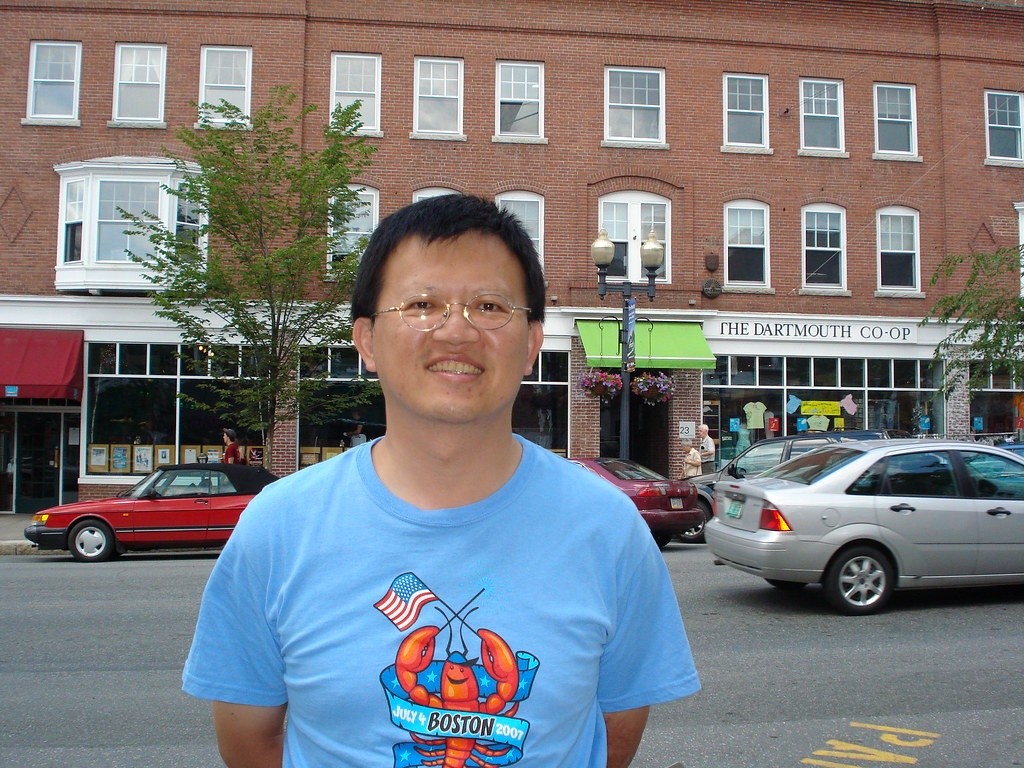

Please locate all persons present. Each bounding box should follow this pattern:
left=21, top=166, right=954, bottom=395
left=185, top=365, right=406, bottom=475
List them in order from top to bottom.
left=182, top=193, right=702, bottom=768
left=347, top=412, right=367, bottom=446
left=735, top=415, right=754, bottom=459
left=696, top=424, right=716, bottom=474
left=222, top=428, right=240, bottom=464
left=677, top=438, right=702, bottom=480
left=923, top=456, right=941, bottom=478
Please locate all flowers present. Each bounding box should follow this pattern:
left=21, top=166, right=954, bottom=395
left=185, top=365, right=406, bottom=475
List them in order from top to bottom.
left=577, top=371, right=621, bottom=403
left=630, top=372, right=677, bottom=406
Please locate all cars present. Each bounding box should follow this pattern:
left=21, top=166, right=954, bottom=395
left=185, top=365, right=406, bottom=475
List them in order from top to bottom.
left=703, top=436, right=1024, bottom=619
left=995, top=443, right=1024, bottom=457
left=565, top=455, right=703, bottom=547
left=23, top=460, right=283, bottom=564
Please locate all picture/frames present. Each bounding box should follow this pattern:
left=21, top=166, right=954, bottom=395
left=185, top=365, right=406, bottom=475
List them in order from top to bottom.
left=88, top=444, right=108, bottom=473
left=110, top=444, right=131, bottom=473
left=133, top=445, right=153, bottom=474
left=155, top=445, right=174, bottom=469
left=180, top=445, right=201, bottom=465
left=203, top=446, right=223, bottom=463
left=225, top=446, right=244, bottom=464
left=247, top=446, right=265, bottom=468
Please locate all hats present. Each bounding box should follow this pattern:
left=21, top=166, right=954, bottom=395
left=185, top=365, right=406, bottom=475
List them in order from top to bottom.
left=223, top=428, right=236, bottom=438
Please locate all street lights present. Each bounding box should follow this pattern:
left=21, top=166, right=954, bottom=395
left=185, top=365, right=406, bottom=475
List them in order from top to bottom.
left=589, top=225, right=665, bottom=460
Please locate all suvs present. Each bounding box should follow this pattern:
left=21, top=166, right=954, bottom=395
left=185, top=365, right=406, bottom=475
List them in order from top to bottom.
left=673, top=427, right=917, bottom=546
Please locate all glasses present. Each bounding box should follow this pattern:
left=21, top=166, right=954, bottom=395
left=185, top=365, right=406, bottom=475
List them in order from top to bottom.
left=369, top=293, right=531, bottom=332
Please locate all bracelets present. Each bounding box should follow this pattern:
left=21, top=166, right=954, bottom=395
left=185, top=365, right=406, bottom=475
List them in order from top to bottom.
left=701, top=452, right=703, bottom=456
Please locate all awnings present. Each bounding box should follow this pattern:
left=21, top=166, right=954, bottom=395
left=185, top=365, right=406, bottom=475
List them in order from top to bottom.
left=576, top=319, right=717, bottom=370
left=0, top=328, right=82, bottom=403
